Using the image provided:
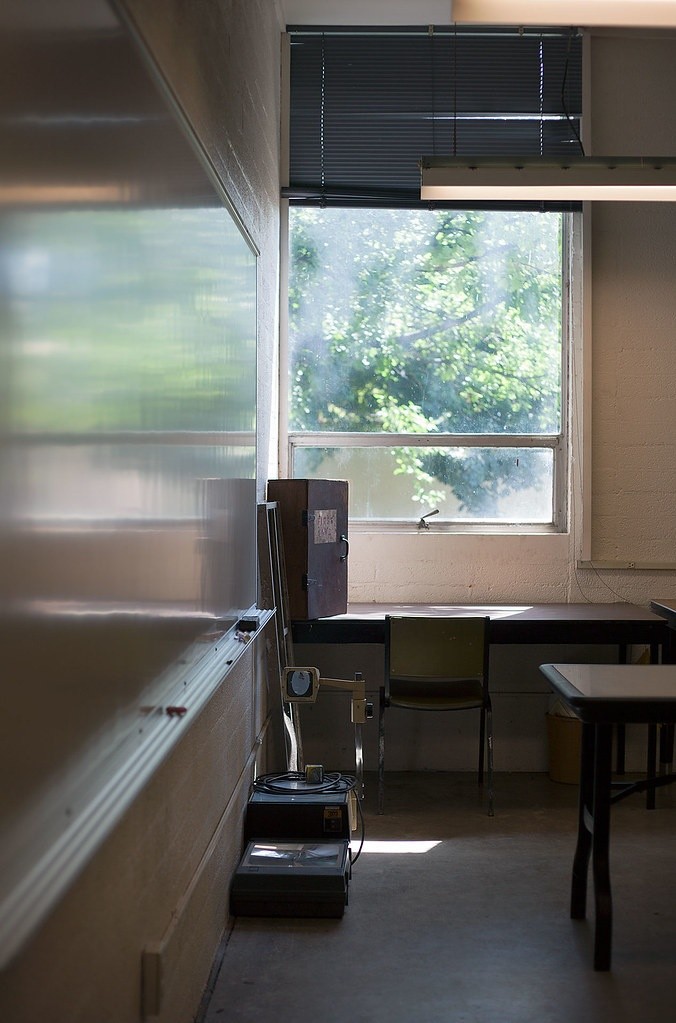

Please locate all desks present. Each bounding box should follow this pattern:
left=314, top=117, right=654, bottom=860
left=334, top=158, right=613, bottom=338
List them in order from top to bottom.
left=650, top=599, right=676, bottom=779
left=289, top=600, right=671, bottom=811
left=538, top=663, right=676, bottom=973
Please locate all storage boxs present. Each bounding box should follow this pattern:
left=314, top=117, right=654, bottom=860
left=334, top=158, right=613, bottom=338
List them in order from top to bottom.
left=267, top=479, right=351, bottom=622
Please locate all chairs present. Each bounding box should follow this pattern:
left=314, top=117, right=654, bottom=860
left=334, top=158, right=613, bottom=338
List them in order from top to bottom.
left=378, top=614, right=495, bottom=816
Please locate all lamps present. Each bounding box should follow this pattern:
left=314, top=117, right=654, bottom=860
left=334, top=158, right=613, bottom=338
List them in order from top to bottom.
left=416, top=23, right=676, bottom=202
